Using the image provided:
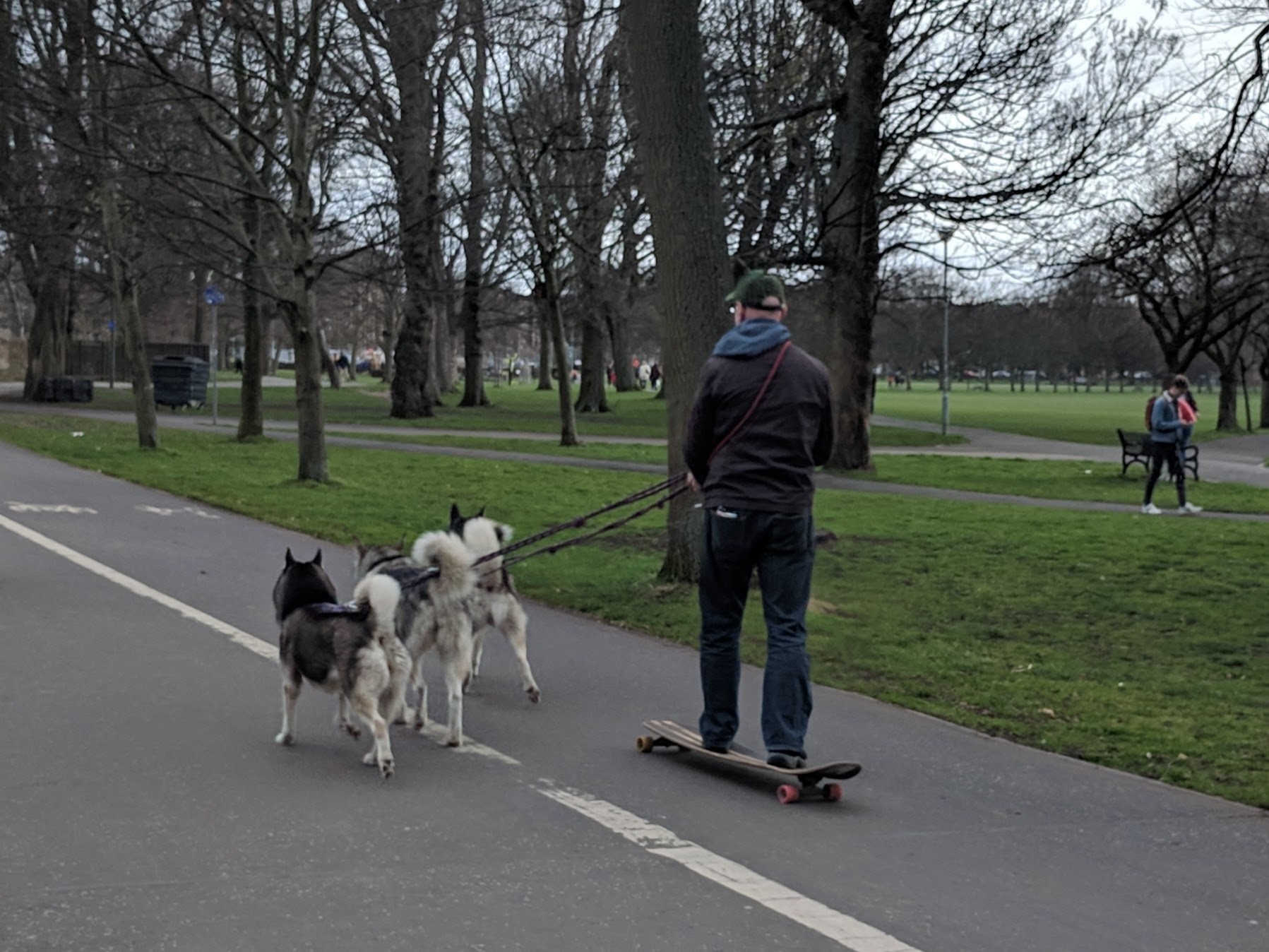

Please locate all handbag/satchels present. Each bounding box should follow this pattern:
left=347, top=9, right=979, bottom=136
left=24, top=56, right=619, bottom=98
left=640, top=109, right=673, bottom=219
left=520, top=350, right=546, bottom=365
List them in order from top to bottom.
left=1178, top=397, right=1197, bottom=422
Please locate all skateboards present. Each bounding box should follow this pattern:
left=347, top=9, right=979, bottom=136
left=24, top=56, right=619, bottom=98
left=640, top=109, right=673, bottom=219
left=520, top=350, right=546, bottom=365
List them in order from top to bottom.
left=634, top=720, right=862, bottom=806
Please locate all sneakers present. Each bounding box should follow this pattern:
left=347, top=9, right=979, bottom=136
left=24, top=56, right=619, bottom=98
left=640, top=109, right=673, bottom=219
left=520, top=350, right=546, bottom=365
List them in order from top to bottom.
left=1142, top=503, right=1161, bottom=514
left=1178, top=503, right=1203, bottom=514
left=703, top=740, right=734, bottom=755
left=766, top=751, right=806, bottom=769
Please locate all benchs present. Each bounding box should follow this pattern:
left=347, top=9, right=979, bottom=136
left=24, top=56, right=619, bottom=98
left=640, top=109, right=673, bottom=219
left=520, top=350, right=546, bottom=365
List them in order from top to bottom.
left=1118, top=430, right=1200, bottom=481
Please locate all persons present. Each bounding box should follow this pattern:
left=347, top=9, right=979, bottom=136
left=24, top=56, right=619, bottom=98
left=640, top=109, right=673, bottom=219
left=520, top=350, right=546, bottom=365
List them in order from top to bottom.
left=682, top=273, right=837, bottom=770
left=333, top=350, right=350, bottom=378
left=235, top=357, right=243, bottom=374
left=607, top=360, right=662, bottom=390
left=894, top=368, right=905, bottom=387
left=1141, top=375, right=1204, bottom=514
left=1162, top=380, right=1198, bottom=484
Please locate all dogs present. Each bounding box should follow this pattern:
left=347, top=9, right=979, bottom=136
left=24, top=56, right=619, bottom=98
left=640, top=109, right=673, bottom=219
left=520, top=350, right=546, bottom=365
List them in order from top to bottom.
left=354, top=500, right=541, bottom=746
left=272, top=545, right=413, bottom=777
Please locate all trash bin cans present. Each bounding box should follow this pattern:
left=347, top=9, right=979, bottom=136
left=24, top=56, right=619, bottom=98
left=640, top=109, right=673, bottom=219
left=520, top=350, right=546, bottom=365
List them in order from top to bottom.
left=151, top=354, right=211, bottom=408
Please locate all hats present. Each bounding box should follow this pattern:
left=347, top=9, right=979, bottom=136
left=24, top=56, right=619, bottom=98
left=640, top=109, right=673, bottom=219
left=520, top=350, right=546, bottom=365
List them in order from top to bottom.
left=724, top=271, right=784, bottom=311
